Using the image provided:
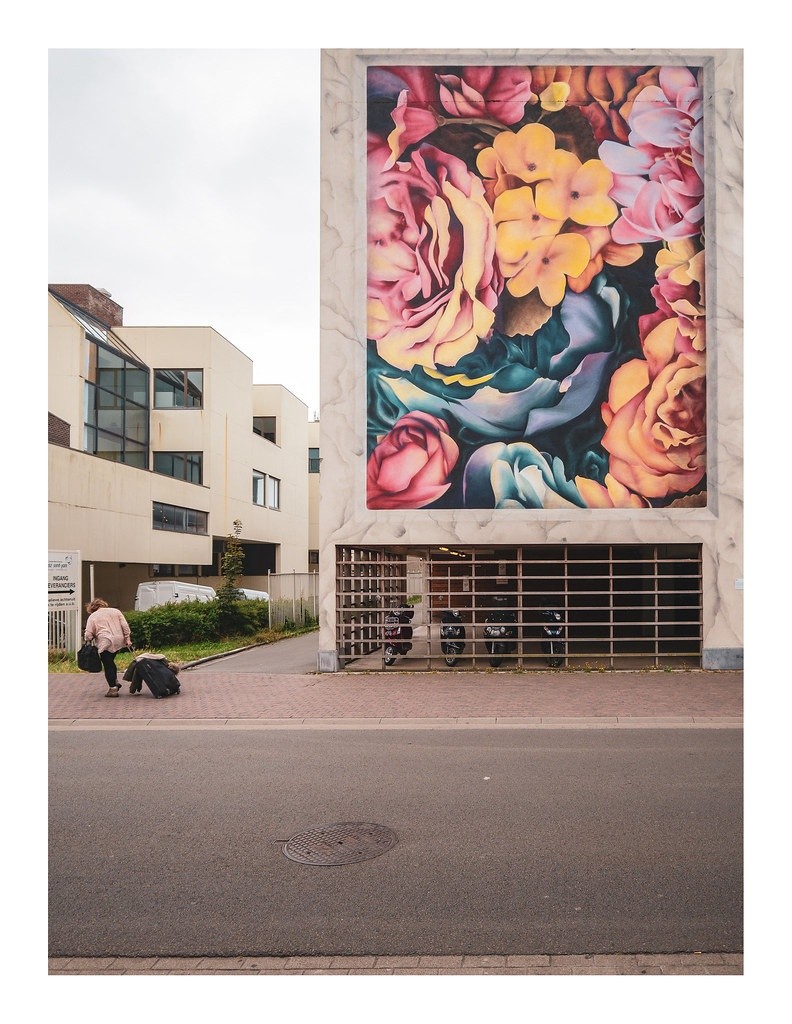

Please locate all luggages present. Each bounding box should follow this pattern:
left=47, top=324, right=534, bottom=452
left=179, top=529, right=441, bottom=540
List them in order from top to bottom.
left=126, top=644, right=181, bottom=699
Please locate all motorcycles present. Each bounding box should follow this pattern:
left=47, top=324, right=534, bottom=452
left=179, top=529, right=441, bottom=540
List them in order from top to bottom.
left=383, top=603, right=414, bottom=666
left=541, top=610, right=566, bottom=667
left=440, top=611, right=467, bottom=666
left=482, top=611, right=518, bottom=667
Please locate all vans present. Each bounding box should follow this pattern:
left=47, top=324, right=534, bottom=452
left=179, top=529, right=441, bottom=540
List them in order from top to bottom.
left=134, top=580, right=220, bottom=611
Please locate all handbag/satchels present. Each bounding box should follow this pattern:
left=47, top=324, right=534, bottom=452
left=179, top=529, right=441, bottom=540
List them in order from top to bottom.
left=77, top=641, right=102, bottom=672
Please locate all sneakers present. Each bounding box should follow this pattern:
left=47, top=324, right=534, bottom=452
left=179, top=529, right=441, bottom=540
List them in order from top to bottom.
left=114, top=682, right=122, bottom=690
left=104, top=686, right=119, bottom=697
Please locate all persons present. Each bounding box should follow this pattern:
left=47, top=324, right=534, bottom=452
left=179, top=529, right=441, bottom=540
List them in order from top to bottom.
left=84, top=599, right=132, bottom=698
